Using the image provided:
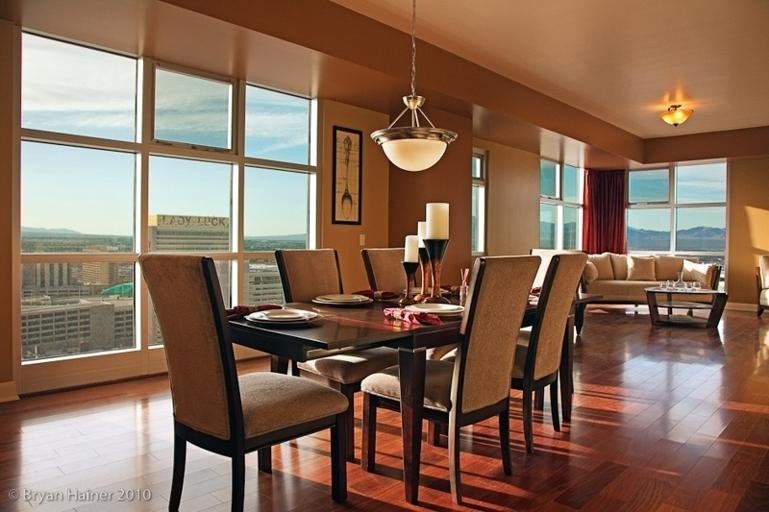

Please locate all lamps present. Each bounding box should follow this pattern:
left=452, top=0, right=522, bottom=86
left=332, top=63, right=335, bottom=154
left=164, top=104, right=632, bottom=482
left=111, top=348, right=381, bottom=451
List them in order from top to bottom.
left=370, top=0, right=456, bottom=172
left=661, top=105, right=694, bottom=127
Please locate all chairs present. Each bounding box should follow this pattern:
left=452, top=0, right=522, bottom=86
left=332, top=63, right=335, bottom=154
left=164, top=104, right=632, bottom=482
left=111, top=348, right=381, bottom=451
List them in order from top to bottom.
left=275, top=248, right=399, bottom=464
left=361, top=248, right=457, bottom=446
left=518, top=249, right=581, bottom=411
left=428, top=251, right=588, bottom=455
left=138, top=254, right=348, bottom=512
left=360, top=254, right=542, bottom=504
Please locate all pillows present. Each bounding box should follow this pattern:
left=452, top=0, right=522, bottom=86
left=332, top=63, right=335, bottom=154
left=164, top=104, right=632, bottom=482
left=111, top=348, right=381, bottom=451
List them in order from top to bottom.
left=626, top=256, right=655, bottom=281
left=680, top=259, right=716, bottom=286
left=584, top=261, right=598, bottom=285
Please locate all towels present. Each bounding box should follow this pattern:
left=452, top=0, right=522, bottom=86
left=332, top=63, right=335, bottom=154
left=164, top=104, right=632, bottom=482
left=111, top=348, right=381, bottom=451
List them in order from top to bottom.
left=440, top=283, right=460, bottom=293
left=352, top=289, right=400, bottom=302
left=224, top=304, right=284, bottom=319
left=383, top=306, right=445, bottom=326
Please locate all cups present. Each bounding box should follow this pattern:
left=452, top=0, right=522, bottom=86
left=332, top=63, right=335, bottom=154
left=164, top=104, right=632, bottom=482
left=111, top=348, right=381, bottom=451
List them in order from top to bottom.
left=666, top=279, right=675, bottom=290
left=660, top=281, right=667, bottom=289
left=686, top=282, right=693, bottom=291
left=693, top=282, right=701, bottom=291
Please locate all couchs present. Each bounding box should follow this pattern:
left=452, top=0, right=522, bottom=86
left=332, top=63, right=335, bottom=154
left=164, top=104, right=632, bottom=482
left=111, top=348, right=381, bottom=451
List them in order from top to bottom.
left=581, top=252, right=721, bottom=318
left=755, top=255, right=769, bottom=318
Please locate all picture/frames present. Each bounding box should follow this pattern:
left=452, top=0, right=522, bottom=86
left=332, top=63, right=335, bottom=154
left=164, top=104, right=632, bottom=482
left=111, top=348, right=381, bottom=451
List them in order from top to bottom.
left=331, top=124, right=363, bottom=224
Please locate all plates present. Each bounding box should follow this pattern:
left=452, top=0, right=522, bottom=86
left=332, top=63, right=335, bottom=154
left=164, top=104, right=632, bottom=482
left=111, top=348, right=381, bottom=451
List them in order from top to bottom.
left=403, top=286, right=448, bottom=295
left=312, top=292, right=374, bottom=306
left=406, top=303, right=465, bottom=318
left=244, top=309, right=323, bottom=326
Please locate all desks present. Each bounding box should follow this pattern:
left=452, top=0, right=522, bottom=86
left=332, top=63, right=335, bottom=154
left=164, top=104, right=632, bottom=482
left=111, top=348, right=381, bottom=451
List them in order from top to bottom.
left=643, top=286, right=728, bottom=330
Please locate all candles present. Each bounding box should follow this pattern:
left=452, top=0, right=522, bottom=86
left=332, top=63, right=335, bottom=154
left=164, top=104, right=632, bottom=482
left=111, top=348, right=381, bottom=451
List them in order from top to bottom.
left=426, top=203, right=449, bottom=238
left=418, top=221, right=426, bottom=247
left=405, top=235, right=419, bottom=261
